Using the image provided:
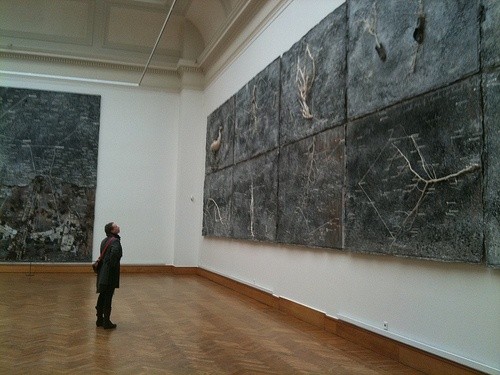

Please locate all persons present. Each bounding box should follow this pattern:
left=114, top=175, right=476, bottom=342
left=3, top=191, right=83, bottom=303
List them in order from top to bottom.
left=96, top=222, right=123, bottom=329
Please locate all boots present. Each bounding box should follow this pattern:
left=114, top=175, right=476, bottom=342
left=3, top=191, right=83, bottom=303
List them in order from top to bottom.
left=96, top=309, right=117, bottom=329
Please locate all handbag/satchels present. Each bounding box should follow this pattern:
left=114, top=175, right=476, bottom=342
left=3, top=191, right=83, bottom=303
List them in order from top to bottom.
left=92, top=260, right=99, bottom=272
